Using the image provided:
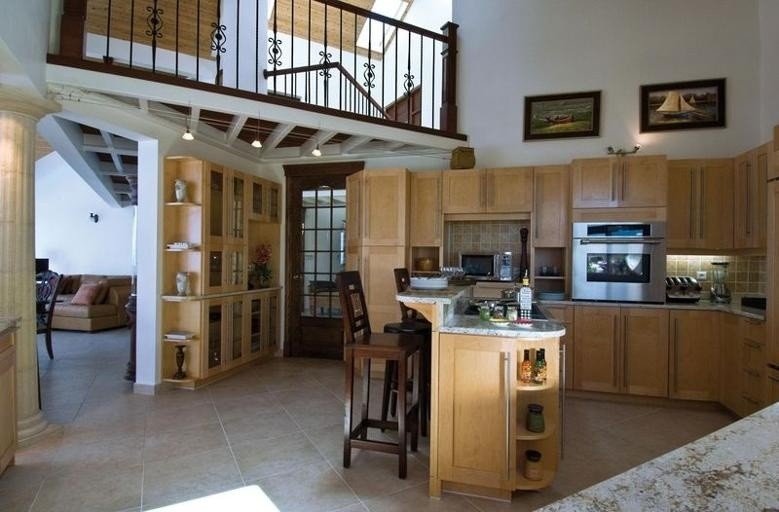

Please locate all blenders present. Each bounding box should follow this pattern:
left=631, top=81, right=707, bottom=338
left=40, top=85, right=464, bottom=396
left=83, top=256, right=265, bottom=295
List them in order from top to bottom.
left=710, top=261, right=732, bottom=304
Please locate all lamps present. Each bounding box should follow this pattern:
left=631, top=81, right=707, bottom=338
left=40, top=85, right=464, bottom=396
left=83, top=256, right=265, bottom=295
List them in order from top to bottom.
left=182, top=106, right=321, bottom=157
left=90, top=213, right=98, bottom=223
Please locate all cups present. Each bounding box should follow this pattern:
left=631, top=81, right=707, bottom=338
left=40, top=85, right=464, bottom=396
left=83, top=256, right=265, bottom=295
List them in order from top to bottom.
left=539, top=264, right=561, bottom=276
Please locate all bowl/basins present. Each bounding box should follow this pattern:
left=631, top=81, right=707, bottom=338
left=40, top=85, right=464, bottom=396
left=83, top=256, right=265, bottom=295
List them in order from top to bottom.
left=415, top=256, right=436, bottom=270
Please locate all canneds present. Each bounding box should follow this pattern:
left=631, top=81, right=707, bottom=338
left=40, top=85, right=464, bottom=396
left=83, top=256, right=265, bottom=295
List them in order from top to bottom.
left=480, top=306, right=490, bottom=320
left=493, top=306, right=503, bottom=319
left=506, top=306, right=518, bottom=321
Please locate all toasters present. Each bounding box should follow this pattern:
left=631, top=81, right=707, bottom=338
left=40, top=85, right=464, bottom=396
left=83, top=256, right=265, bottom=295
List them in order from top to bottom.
left=666, top=276, right=703, bottom=303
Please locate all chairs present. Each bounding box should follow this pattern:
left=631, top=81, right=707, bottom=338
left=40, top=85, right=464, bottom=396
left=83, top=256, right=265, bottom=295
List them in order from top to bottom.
left=36, top=270, right=63, bottom=359
left=308, top=281, right=342, bottom=315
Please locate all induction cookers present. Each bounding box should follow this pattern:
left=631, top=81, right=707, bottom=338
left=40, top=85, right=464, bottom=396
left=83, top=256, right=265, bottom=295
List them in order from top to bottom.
left=464, top=298, right=548, bottom=322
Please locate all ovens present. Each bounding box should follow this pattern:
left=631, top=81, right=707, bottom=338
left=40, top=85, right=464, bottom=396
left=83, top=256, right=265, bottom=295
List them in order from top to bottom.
left=572, top=220, right=666, bottom=303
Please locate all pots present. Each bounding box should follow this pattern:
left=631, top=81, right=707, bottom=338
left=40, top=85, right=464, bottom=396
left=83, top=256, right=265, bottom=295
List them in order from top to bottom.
left=501, top=287, right=517, bottom=299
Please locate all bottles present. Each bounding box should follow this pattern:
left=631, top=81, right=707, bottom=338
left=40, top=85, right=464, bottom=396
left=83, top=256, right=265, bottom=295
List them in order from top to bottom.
left=174, top=178, right=186, bottom=201
left=175, top=271, right=188, bottom=297
left=519, top=276, right=533, bottom=320
left=480, top=306, right=517, bottom=322
left=521, top=347, right=548, bottom=384
left=526, top=403, right=545, bottom=433
left=524, top=450, right=544, bottom=481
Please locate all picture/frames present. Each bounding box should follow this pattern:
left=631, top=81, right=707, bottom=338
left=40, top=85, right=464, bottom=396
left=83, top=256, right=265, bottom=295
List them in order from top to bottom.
left=639, top=78, right=727, bottom=133
left=524, top=91, right=600, bottom=140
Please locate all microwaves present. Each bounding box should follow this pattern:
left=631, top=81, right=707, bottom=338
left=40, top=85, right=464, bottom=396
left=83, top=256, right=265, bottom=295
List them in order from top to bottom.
left=458, top=250, right=516, bottom=282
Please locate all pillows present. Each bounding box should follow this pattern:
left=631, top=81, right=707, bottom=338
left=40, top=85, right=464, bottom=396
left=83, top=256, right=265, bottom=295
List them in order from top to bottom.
left=70, top=283, right=112, bottom=305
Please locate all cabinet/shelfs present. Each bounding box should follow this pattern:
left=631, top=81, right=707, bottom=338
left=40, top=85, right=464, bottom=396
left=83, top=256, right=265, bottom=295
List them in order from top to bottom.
left=161, top=156, right=282, bottom=391
left=0, top=316, right=21, bottom=476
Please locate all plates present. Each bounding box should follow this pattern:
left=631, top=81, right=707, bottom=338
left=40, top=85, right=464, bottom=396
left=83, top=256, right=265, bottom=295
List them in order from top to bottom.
left=410, top=277, right=449, bottom=290
left=537, top=289, right=565, bottom=300
left=489, top=318, right=534, bottom=329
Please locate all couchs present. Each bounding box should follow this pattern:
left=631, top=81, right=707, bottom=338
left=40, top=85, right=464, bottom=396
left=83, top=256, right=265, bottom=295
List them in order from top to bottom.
left=51, top=274, right=137, bottom=333
